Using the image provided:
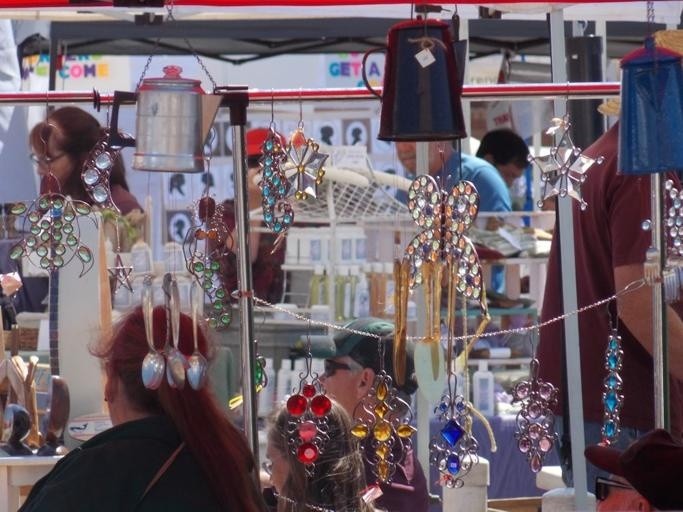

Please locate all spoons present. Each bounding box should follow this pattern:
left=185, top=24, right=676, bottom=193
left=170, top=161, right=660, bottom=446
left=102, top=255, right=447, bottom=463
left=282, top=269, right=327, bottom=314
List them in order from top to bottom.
left=139, top=274, right=165, bottom=391
left=166, top=278, right=189, bottom=391
left=187, top=278, right=210, bottom=395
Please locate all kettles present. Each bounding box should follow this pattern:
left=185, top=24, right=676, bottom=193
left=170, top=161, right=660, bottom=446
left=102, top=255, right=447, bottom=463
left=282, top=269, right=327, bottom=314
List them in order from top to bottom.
left=361, top=16, right=469, bottom=144
left=615, top=36, right=683, bottom=180
left=105, top=64, right=224, bottom=174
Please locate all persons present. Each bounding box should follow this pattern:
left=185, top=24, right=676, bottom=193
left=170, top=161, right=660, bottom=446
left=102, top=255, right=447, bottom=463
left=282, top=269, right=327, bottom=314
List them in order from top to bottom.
left=28, top=106, right=145, bottom=251
left=538, top=112, right=683, bottom=484
left=395, top=121, right=520, bottom=310
left=209, top=126, right=294, bottom=305
left=473, top=130, right=531, bottom=321
left=16, top=304, right=258, bottom=512
left=316, top=319, right=429, bottom=512
left=265, top=396, right=365, bottom=512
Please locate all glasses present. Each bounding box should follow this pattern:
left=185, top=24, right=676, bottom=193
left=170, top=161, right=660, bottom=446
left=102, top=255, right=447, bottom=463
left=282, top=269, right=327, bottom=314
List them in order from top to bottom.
left=29, top=151, right=65, bottom=166
left=324, top=358, right=352, bottom=376
left=595, top=477, right=635, bottom=501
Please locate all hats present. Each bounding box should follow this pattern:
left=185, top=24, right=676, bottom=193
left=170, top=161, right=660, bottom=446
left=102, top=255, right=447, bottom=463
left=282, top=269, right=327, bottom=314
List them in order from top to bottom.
left=596, top=29, right=683, bottom=117
left=301, top=318, right=420, bottom=394
